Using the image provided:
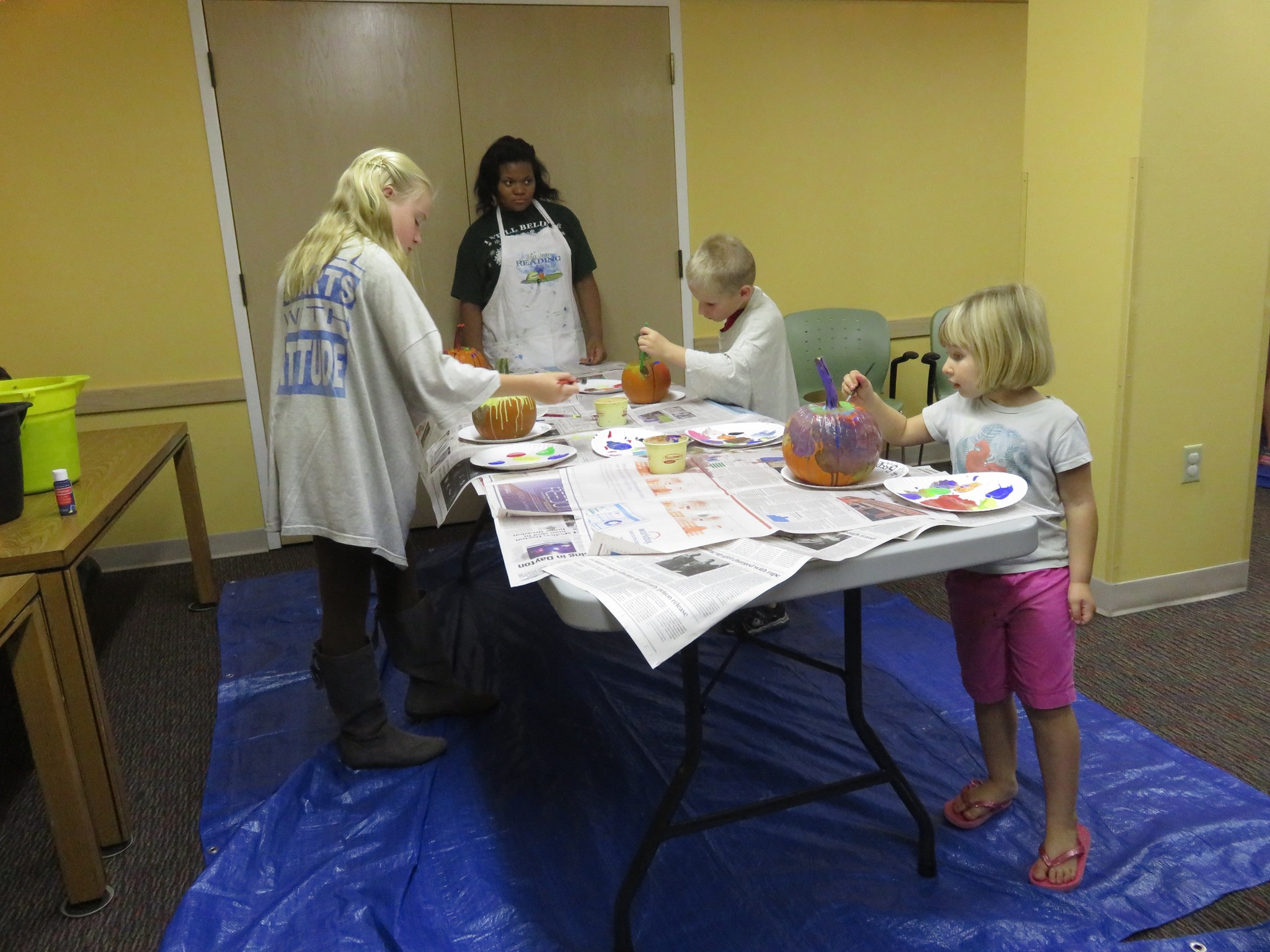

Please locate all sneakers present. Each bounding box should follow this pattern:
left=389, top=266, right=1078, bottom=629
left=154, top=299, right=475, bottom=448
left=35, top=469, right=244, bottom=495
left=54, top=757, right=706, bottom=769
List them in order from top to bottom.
left=724, top=602, right=789, bottom=635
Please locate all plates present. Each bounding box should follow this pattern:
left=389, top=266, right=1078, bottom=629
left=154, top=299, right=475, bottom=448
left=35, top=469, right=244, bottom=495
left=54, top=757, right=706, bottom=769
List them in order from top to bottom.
left=576, top=379, right=624, bottom=394
left=610, top=389, right=685, bottom=408
left=458, top=420, right=552, bottom=443
left=685, top=422, right=786, bottom=448
left=591, top=426, right=665, bottom=458
left=470, top=443, right=577, bottom=470
left=781, top=459, right=909, bottom=490
left=884, top=472, right=1029, bottom=513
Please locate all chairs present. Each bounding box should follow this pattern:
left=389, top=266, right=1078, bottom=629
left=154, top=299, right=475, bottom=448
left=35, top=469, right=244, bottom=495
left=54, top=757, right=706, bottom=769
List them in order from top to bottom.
left=919, top=305, right=962, bottom=469
left=780, top=308, right=919, bottom=467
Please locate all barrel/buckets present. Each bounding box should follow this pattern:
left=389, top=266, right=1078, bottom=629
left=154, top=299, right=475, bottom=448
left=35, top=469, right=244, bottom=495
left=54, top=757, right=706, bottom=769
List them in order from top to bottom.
left=593, top=397, right=629, bottom=428
left=643, top=433, right=690, bottom=474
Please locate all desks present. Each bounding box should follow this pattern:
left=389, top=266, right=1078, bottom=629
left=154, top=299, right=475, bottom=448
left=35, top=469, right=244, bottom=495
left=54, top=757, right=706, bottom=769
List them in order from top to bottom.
left=415, top=358, right=1040, bottom=952
left=1, top=422, right=235, bottom=853
left=2, top=572, right=116, bottom=918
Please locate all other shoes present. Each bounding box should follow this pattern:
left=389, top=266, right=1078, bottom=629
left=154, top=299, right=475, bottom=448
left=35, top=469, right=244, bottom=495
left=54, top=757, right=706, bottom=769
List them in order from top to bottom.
left=405, top=683, right=495, bottom=719
left=338, top=724, right=447, bottom=768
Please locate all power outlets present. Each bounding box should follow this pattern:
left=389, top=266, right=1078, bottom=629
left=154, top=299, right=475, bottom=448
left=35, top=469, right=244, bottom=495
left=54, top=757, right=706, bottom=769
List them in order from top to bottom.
left=1181, top=443, right=1201, bottom=485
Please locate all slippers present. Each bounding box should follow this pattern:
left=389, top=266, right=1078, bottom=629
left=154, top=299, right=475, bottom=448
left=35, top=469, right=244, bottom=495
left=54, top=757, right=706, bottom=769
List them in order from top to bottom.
left=944, top=779, right=1012, bottom=828
left=1029, top=823, right=1090, bottom=891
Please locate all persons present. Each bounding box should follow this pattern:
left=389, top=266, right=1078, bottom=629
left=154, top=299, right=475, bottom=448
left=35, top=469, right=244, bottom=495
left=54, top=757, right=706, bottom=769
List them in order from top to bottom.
left=449, top=133, right=609, bottom=367
left=261, top=145, right=586, bottom=776
left=636, top=232, right=806, bottom=427
left=839, top=280, right=1102, bottom=892
